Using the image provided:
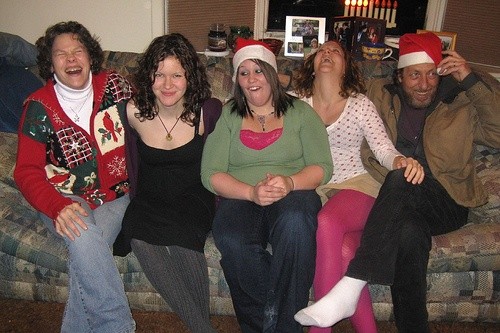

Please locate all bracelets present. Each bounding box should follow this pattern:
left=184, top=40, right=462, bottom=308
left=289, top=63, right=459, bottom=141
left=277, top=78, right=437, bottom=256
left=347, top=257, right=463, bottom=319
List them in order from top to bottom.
left=288, top=176, right=295, bottom=191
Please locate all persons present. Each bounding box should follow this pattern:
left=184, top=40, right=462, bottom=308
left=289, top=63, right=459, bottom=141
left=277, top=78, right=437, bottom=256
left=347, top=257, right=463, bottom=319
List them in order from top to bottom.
left=13, top=20, right=139, bottom=333
left=289, top=23, right=317, bottom=54
left=112, top=32, right=222, bottom=333
left=294, top=34, right=500, bottom=333
left=199, top=39, right=334, bottom=333
left=286, top=39, right=424, bottom=333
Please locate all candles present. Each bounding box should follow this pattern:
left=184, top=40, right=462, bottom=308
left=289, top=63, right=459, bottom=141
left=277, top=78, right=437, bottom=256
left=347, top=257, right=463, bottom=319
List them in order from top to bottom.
left=344, top=0, right=398, bottom=28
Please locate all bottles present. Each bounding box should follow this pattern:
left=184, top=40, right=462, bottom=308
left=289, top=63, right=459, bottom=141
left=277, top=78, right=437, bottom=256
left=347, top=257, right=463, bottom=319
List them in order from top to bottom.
left=208, top=23, right=227, bottom=51
left=227, top=26, right=253, bottom=49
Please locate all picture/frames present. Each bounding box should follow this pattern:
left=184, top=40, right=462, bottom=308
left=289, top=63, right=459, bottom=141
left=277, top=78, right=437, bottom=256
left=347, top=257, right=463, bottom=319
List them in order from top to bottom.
left=284, top=16, right=326, bottom=60
left=329, top=16, right=354, bottom=53
left=355, top=16, right=387, bottom=44
left=417, top=30, right=457, bottom=51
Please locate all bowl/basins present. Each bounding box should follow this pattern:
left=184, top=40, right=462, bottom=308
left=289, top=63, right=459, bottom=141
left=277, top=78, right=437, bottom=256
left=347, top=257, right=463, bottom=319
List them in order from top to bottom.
left=257, top=38, right=284, bottom=56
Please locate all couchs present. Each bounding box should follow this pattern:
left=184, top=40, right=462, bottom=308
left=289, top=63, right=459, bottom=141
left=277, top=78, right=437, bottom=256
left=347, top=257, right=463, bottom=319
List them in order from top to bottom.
left=0, top=50, right=500, bottom=324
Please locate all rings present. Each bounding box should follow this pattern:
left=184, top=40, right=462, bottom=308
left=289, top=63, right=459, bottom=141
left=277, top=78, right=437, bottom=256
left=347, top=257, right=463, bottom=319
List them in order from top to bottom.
left=453, top=61, right=456, bottom=67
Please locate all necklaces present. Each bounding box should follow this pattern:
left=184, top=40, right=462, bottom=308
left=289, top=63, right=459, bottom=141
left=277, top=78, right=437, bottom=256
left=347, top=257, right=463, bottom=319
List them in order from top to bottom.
left=56, top=91, right=88, bottom=123
left=250, top=110, right=274, bottom=132
left=154, top=111, right=180, bottom=141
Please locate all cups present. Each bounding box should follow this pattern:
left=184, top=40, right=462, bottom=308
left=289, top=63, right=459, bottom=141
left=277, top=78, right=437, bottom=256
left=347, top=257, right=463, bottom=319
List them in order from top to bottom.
left=361, top=42, right=392, bottom=61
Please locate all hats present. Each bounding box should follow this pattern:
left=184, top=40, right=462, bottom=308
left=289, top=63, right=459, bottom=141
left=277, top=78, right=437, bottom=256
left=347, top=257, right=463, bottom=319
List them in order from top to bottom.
left=397, top=31, right=449, bottom=76
left=230, top=34, right=278, bottom=83
